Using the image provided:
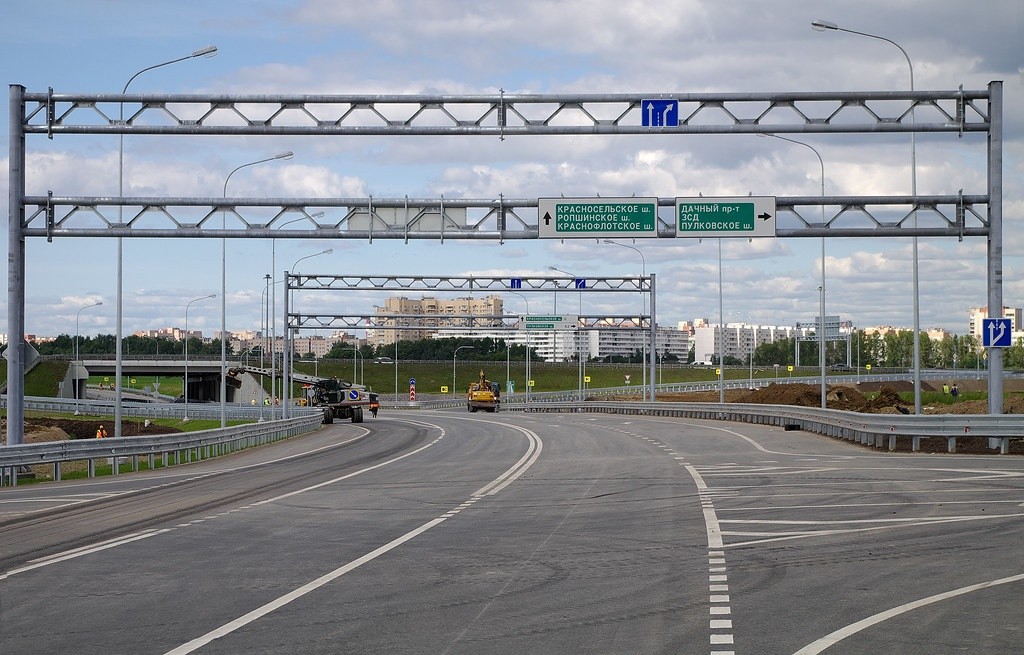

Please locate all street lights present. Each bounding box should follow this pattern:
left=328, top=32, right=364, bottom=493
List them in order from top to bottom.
left=810, top=21, right=920, bottom=414
left=115, top=43, right=219, bottom=435
left=758, top=132, right=827, bottom=409
left=221, top=150, right=296, bottom=430
left=258, top=210, right=335, bottom=421
left=604, top=241, right=646, bottom=402
left=548, top=267, right=582, bottom=402
left=143, top=293, right=217, bottom=423
left=75, top=301, right=102, bottom=412
left=454, top=346, right=475, bottom=400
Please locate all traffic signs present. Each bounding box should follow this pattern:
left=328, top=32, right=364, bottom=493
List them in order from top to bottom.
left=538, top=198, right=658, bottom=239
left=676, top=198, right=776, bottom=238
left=518, top=315, right=578, bottom=330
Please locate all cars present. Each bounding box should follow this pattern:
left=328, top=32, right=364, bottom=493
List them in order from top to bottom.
left=342, top=348, right=364, bottom=385
left=374, top=357, right=395, bottom=364
left=172, top=396, right=185, bottom=404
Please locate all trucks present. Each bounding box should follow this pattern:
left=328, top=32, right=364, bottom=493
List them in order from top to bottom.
left=465, top=385, right=497, bottom=413
left=317, top=387, right=371, bottom=423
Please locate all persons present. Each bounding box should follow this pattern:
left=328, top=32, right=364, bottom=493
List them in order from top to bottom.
left=99, top=382, right=103, bottom=390
left=942, top=383, right=950, bottom=396
left=951, top=384, right=959, bottom=396
left=264, top=397, right=271, bottom=407
left=274, top=398, right=279, bottom=407
left=252, top=399, right=256, bottom=404
left=371, top=404, right=380, bottom=418
left=97, top=425, right=107, bottom=439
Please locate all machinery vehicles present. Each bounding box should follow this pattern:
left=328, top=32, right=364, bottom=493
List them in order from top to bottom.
left=470, top=369, right=495, bottom=401
left=297, top=386, right=318, bottom=407
